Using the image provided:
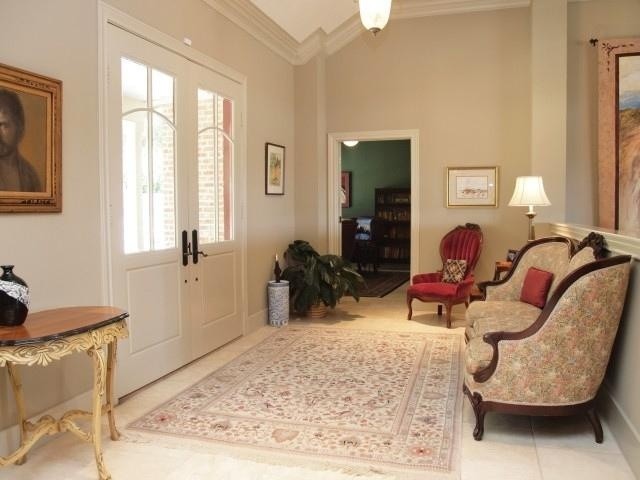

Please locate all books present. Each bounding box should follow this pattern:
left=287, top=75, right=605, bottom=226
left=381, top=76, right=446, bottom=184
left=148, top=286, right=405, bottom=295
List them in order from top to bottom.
left=375, top=192, right=410, bottom=260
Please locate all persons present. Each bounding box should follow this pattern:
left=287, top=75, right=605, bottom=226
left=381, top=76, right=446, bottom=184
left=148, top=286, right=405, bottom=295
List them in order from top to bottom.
left=1, top=86, right=46, bottom=192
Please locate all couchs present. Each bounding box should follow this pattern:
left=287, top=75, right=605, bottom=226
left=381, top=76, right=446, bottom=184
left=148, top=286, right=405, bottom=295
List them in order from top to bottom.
left=463, top=232, right=632, bottom=443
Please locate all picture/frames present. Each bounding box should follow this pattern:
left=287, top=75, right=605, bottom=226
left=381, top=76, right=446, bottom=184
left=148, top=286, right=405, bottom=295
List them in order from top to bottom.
left=594, top=37, right=639, bottom=231
left=0, top=63, right=63, bottom=214
left=264, top=142, right=286, bottom=196
left=445, top=165, right=501, bottom=208
left=341, top=170, right=352, bottom=209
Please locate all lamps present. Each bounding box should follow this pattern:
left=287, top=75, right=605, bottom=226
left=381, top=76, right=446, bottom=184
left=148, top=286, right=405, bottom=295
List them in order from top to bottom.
left=358, top=0, right=392, bottom=37
left=507, top=175, right=552, bottom=243
left=0, top=305, right=130, bottom=480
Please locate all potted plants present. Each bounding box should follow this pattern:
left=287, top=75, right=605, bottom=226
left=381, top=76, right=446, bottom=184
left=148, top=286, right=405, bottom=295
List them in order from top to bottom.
left=279, top=240, right=368, bottom=319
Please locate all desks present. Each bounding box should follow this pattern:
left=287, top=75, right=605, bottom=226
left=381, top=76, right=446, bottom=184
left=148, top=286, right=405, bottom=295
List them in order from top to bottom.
left=494, top=258, right=513, bottom=282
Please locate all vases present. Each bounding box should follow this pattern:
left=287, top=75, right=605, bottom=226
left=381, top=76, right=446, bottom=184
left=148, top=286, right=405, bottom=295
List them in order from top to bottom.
left=0, top=265, right=30, bottom=327
left=268, top=278, right=289, bottom=328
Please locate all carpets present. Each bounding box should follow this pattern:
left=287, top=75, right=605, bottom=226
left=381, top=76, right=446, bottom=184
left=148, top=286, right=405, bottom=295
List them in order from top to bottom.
left=342, top=267, right=410, bottom=300
left=117, top=325, right=465, bottom=479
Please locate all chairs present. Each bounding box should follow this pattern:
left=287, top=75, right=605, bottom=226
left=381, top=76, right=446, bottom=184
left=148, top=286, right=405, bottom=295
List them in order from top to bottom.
left=349, top=218, right=378, bottom=272
left=406, top=223, right=486, bottom=328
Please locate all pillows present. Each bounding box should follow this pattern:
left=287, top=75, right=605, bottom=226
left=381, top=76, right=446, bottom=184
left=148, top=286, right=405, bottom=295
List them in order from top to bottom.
left=441, top=260, right=470, bottom=284
left=519, top=267, right=555, bottom=310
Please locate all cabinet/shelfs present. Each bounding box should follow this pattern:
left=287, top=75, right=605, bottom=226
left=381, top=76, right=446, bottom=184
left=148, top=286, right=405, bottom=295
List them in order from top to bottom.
left=374, top=187, right=411, bottom=263
left=342, top=220, right=383, bottom=262
